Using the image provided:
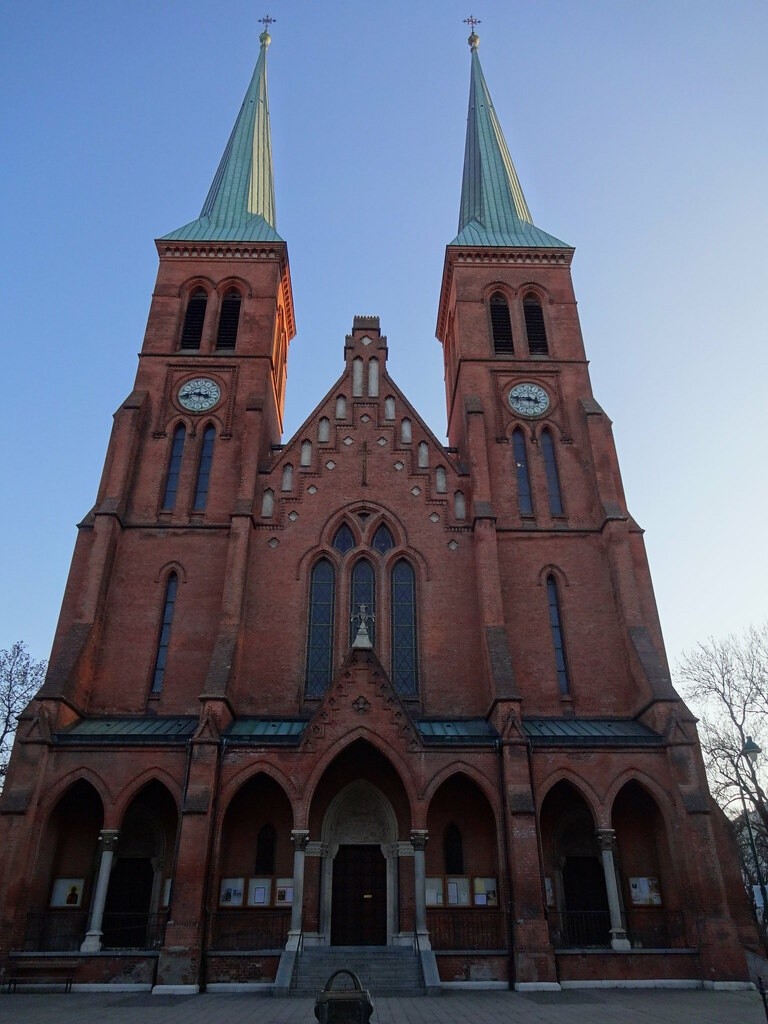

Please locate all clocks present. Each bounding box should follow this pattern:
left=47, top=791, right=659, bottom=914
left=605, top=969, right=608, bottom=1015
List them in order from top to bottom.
left=177, top=377, right=221, bottom=412
left=508, top=383, right=549, bottom=416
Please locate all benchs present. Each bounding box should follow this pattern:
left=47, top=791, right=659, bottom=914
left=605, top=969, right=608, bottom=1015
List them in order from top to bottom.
left=4, top=959, right=81, bottom=995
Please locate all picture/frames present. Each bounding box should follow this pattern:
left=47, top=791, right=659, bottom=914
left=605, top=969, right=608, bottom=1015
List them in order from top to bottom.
left=159, top=873, right=173, bottom=910
left=424, top=873, right=500, bottom=910
left=626, top=873, right=664, bottom=908
left=217, top=874, right=294, bottom=909
left=544, top=874, right=557, bottom=909
left=46, top=876, right=88, bottom=911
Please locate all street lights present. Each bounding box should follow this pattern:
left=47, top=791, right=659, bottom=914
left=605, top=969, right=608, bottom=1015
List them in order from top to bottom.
left=734, top=736, right=768, bottom=906
